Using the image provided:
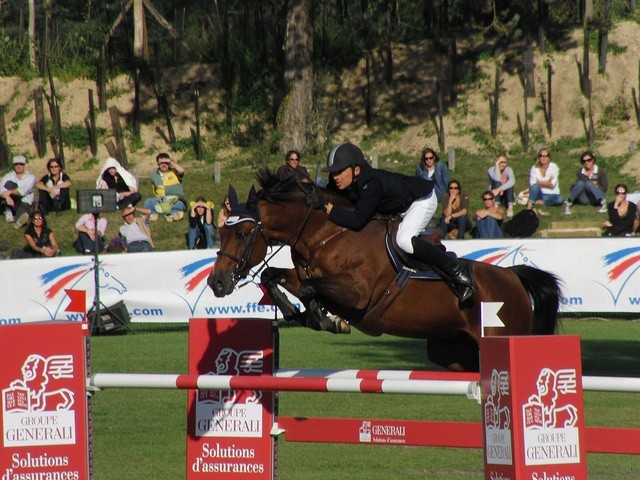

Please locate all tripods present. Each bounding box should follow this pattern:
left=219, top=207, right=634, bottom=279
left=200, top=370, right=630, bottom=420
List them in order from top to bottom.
left=81, top=213, right=136, bottom=337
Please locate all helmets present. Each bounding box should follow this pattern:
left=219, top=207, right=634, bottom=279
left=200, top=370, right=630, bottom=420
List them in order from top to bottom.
left=321, top=143, right=364, bottom=173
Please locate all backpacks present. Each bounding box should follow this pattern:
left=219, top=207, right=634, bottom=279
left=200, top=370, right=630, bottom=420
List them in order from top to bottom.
left=505, top=209, right=539, bottom=238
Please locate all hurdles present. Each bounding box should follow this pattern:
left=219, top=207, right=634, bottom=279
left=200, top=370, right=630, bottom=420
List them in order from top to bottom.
left=88, top=367, right=640, bottom=454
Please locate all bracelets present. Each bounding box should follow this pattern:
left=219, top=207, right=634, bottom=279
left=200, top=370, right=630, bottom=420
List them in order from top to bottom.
left=450, top=215, right=455, bottom=221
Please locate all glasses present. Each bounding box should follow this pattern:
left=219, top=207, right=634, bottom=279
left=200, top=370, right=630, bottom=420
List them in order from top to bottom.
left=540, top=154, right=549, bottom=157
left=424, top=155, right=434, bottom=160
left=290, top=158, right=299, bottom=161
left=583, top=159, right=592, bottom=163
left=50, top=166, right=59, bottom=168
left=449, top=187, right=459, bottom=189
left=616, top=191, right=626, bottom=194
left=483, top=198, right=493, bottom=200
left=33, top=217, right=43, bottom=220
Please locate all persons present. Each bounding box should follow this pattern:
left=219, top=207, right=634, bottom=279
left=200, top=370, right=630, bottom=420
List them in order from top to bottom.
left=323, top=142, right=476, bottom=303
left=416, top=147, right=450, bottom=202
left=526, top=147, right=563, bottom=210
left=276, top=150, right=310, bottom=182
left=563, top=150, right=608, bottom=215
left=143, top=153, right=188, bottom=223
left=487, top=153, right=515, bottom=218
left=0, top=155, right=39, bottom=229
left=95, top=157, right=142, bottom=210
left=35, top=158, right=73, bottom=215
left=440, top=179, right=472, bottom=239
left=603, top=184, right=637, bottom=237
left=472, top=190, right=504, bottom=238
left=216, top=195, right=231, bottom=227
left=188, top=196, right=218, bottom=249
left=118, top=206, right=155, bottom=252
left=10, top=210, right=60, bottom=257
left=74, top=212, right=108, bottom=254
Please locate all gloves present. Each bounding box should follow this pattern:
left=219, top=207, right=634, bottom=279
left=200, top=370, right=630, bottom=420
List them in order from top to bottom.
left=307, top=191, right=328, bottom=212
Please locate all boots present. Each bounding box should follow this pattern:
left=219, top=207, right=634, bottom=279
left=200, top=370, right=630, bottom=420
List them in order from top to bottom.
left=411, top=236, right=474, bottom=303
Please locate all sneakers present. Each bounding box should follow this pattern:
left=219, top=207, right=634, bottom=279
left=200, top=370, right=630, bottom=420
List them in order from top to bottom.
left=599, top=200, right=608, bottom=213
left=564, top=201, right=572, bottom=215
left=57, top=203, right=62, bottom=216
left=4, top=209, right=15, bottom=223
left=506, top=209, right=513, bottom=217
left=167, top=211, right=184, bottom=222
left=14, top=213, right=29, bottom=229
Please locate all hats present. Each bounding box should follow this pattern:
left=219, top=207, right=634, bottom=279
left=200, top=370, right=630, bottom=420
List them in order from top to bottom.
left=12, top=156, right=26, bottom=164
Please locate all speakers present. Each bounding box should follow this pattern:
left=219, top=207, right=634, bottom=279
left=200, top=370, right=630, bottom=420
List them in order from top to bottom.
left=77, top=189, right=117, bottom=212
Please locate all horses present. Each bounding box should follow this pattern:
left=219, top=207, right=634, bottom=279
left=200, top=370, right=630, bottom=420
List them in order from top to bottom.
left=205, top=162, right=564, bottom=377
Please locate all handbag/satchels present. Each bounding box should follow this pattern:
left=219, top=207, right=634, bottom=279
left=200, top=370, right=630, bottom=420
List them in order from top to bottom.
left=516, top=187, right=529, bottom=205
left=107, top=237, right=124, bottom=252
